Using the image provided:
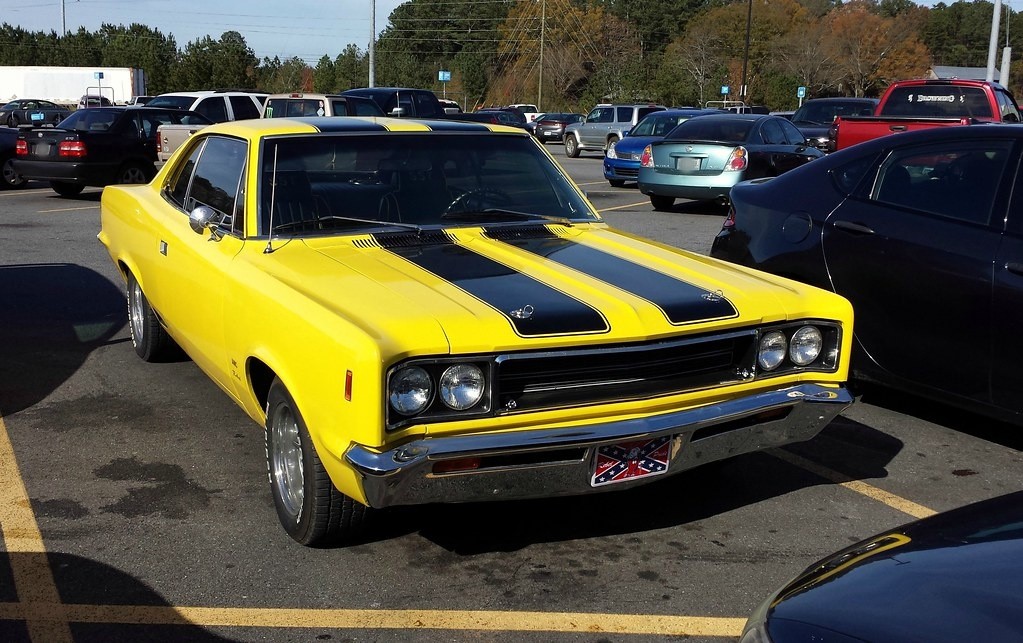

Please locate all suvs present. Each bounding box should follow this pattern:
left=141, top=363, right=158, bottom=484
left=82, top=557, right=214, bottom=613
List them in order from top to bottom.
left=134, top=89, right=271, bottom=129
left=398, top=100, right=463, bottom=113
left=562, top=104, right=673, bottom=157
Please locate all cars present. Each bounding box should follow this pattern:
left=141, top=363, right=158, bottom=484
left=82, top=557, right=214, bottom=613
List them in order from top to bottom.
left=76, top=96, right=115, bottom=112
left=792, top=98, right=881, bottom=155
left=0, top=100, right=71, bottom=189
left=12, top=107, right=222, bottom=196
left=602, top=109, right=732, bottom=186
left=528, top=113, right=587, bottom=143
left=638, top=114, right=825, bottom=212
left=97, top=117, right=854, bottom=545
left=710, top=122, right=1023, bottom=417
left=740, top=492, right=1023, bottom=643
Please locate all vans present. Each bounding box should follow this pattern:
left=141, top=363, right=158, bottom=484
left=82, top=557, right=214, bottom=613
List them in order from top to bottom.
left=125, top=96, right=156, bottom=108
left=724, top=106, right=770, bottom=115
left=769, top=110, right=798, bottom=120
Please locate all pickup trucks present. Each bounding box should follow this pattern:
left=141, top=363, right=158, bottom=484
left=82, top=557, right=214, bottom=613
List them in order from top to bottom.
left=837, top=78, right=1023, bottom=179
left=333, top=87, right=523, bottom=129
left=154, top=91, right=404, bottom=174
left=509, top=104, right=546, bottom=123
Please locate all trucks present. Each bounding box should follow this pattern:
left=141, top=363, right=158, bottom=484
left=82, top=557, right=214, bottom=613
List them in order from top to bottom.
left=0, top=66, right=147, bottom=104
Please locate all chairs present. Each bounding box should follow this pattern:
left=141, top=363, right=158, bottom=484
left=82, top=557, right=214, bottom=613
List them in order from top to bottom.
left=376, top=159, right=477, bottom=223
left=260, top=171, right=321, bottom=235
